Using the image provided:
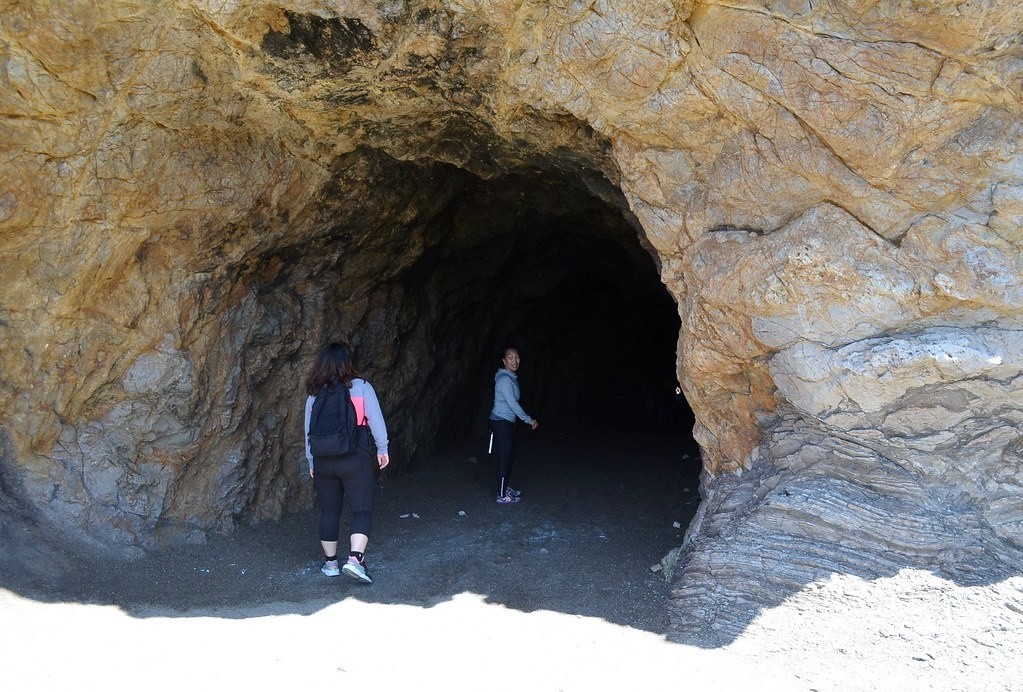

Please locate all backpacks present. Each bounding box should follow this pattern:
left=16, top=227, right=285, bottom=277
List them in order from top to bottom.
left=308, top=377, right=358, bottom=459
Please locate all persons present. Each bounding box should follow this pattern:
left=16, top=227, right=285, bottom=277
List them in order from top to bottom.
left=305, top=342, right=389, bottom=584
left=487, top=347, right=538, bottom=504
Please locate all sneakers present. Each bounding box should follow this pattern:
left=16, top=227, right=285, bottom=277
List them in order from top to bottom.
left=497, top=487, right=521, bottom=496
left=496, top=493, right=521, bottom=503
left=341, top=555, right=372, bottom=583
left=320, top=559, right=340, bottom=576
